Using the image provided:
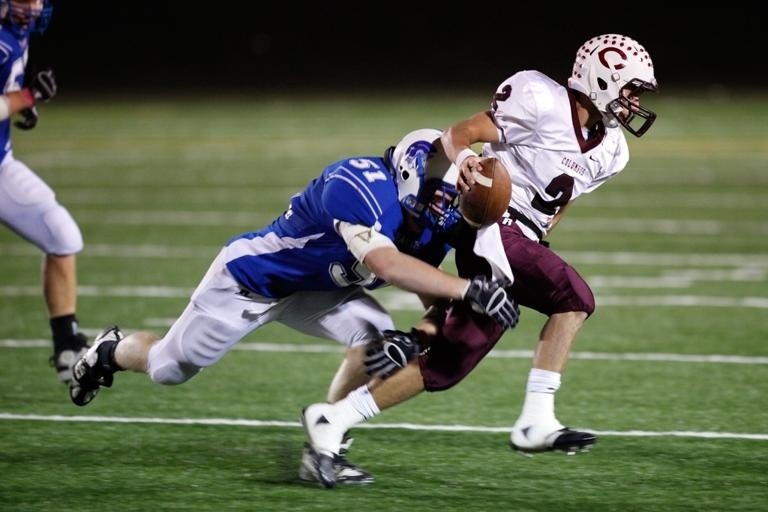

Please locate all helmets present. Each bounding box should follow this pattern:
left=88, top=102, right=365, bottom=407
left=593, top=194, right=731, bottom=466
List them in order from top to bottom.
left=0, top=1, right=54, bottom=39
left=567, top=34, right=658, bottom=137
left=384, top=128, right=462, bottom=244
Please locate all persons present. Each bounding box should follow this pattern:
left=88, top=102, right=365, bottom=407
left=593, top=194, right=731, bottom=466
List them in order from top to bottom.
left=0, top=1, right=94, bottom=385
left=299, top=34, right=660, bottom=490
left=69, top=127, right=523, bottom=486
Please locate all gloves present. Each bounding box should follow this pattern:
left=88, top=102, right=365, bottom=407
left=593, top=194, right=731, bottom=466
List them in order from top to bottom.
left=26, top=68, right=58, bottom=101
left=15, top=106, right=38, bottom=129
left=463, top=276, right=521, bottom=329
left=364, top=327, right=431, bottom=377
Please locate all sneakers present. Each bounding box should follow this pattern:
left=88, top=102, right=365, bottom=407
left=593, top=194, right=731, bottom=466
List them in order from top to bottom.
left=68, top=328, right=122, bottom=406
left=47, top=333, right=97, bottom=384
left=304, top=402, right=348, bottom=488
left=511, top=416, right=597, bottom=457
left=301, top=437, right=374, bottom=486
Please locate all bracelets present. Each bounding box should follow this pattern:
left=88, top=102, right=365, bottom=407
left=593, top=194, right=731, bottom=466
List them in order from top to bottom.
left=454, top=146, right=477, bottom=169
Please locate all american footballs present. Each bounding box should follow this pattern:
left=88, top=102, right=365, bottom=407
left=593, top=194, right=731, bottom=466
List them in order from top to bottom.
left=461, top=159, right=511, bottom=229
